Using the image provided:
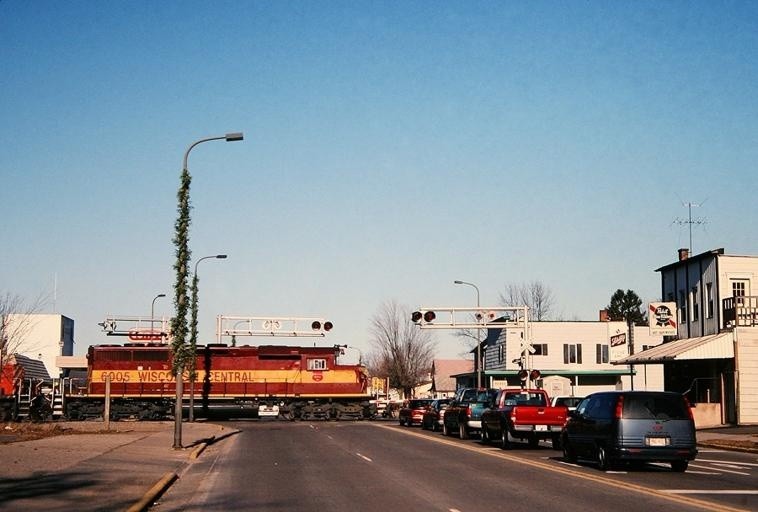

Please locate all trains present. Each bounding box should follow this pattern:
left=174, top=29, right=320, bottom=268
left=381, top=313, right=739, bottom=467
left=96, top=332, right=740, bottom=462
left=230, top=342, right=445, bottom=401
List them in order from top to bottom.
left=0, top=341, right=383, bottom=424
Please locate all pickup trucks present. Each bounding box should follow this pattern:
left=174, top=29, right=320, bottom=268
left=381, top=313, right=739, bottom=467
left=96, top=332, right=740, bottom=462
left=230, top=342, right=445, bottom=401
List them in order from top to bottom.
left=442, top=388, right=499, bottom=441
left=479, top=389, right=569, bottom=450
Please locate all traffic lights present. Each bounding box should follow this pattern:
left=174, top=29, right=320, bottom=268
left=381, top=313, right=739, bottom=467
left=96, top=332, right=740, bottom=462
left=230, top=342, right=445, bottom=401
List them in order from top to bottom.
left=410, top=310, right=436, bottom=325
left=311, top=320, right=335, bottom=332
left=531, top=369, right=541, bottom=382
left=519, top=371, right=528, bottom=381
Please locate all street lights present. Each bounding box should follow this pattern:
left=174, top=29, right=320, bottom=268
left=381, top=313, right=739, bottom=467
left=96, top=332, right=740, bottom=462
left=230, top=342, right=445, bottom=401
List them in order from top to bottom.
left=170, top=130, right=245, bottom=444
left=188, top=254, right=229, bottom=422
left=453, top=280, right=480, bottom=390
left=149, top=294, right=167, bottom=343
left=232, top=320, right=251, bottom=348
left=349, top=346, right=363, bottom=368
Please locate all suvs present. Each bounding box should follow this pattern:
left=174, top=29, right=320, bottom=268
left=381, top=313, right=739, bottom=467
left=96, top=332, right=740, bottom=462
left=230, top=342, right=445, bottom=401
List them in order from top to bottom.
left=560, top=390, right=697, bottom=471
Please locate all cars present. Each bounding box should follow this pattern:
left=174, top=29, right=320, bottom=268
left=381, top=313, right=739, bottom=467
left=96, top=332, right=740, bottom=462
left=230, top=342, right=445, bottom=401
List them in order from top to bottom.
left=549, top=395, right=587, bottom=415
left=399, top=399, right=436, bottom=428
left=423, top=399, right=454, bottom=432
left=255, top=402, right=280, bottom=418
left=383, top=402, right=404, bottom=421
